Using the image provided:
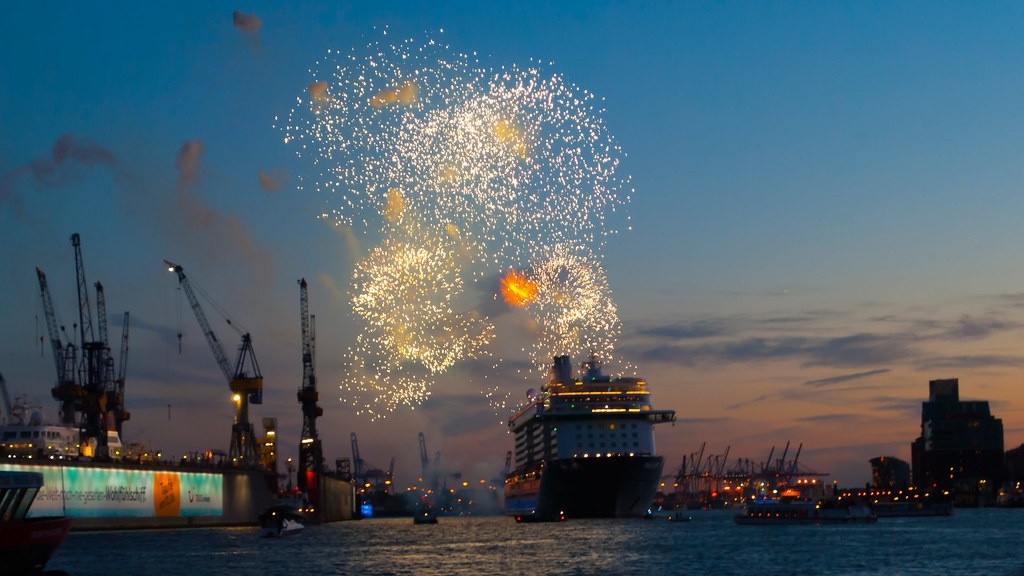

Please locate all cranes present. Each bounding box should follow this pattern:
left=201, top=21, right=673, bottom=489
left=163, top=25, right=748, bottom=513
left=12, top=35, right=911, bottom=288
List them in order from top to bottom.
left=31, top=230, right=132, bottom=444
left=162, top=257, right=264, bottom=465
left=298, top=276, right=326, bottom=494
left=346, top=427, right=512, bottom=493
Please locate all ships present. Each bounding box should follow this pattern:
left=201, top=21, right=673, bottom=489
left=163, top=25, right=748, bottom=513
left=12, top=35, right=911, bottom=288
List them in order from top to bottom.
left=503, top=355, right=676, bottom=520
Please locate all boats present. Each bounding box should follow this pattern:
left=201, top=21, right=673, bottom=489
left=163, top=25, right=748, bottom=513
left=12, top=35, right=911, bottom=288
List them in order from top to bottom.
left=1, top=470, right=72, bottom=575
left=258, top=488, right=311, bottom=537
left=734, top=492, right=878, bottom=525
left=876, top=498, right=955, bottom=517
left=413, top=507, right=437, bottom=524
